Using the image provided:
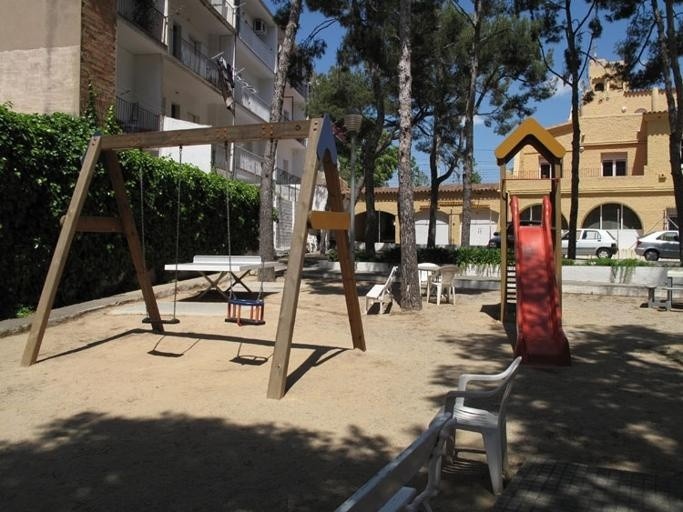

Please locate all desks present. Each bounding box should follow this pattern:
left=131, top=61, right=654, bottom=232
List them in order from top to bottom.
left=163, top=254, right=279, bottom=301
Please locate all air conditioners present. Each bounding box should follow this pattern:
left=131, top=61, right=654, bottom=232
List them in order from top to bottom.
left=253, top=19, right=267, bottom=35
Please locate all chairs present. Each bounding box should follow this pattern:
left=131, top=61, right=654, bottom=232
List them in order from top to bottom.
left=417, top=262, right=459, bottom=307
left=428, top=356, right=523, bottom=496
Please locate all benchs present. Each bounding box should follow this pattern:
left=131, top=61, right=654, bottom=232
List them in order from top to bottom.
left=364, top=266, right=400, bottom=316
left=334, top=409, right=457, bottom=512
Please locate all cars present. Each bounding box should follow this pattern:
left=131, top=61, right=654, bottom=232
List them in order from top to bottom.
left=560, top=228, right=617, bottom=260
left=634, top=230, right=680, bottom=261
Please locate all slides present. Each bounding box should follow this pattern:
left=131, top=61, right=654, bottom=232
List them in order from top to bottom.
left=509, top=195, right=570, bottom=361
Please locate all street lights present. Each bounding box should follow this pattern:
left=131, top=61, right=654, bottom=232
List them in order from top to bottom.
left=338, top=102, right=364, bottom=266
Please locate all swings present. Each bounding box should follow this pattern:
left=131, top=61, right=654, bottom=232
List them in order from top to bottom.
left=225, top=140, right=272, bottom=325
left=139, top=144, right=183, bottom=324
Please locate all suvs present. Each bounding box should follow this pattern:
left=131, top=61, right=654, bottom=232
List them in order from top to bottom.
left=486, top=219, right=541, bottom=250
